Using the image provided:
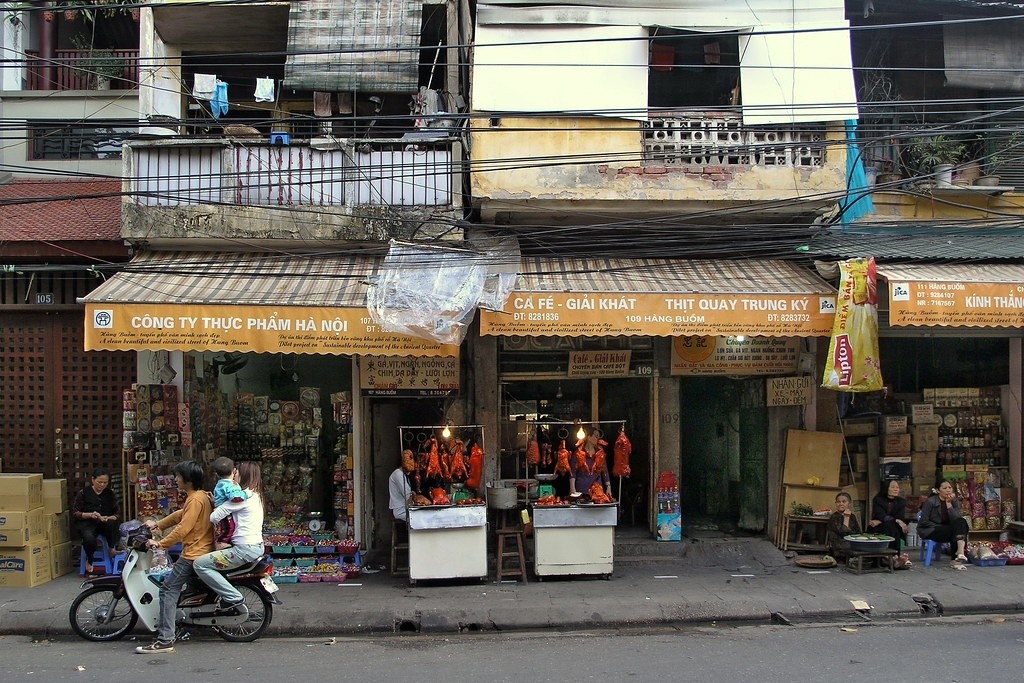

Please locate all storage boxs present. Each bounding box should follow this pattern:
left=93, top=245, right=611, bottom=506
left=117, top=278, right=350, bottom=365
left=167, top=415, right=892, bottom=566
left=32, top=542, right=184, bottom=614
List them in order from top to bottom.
left=844, top=396, right=941, bottom=527
left=0, top=473, right=45, bottom=511
left=42, top=478, right=68, bottom=514
left=0, top=506, right=46, bottom=549
left=45, top=509, right=70, bottom=547
left=263, top=524, right=364, bottom=585
left=0, top=540, right=52, bottom=587
left=49, top=540, right=74, bottom=580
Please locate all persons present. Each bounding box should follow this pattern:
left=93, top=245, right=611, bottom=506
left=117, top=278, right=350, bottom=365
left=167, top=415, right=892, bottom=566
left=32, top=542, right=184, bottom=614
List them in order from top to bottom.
left=568, top=428, right=612, bottom=500
left=214, top=457, right=256, bottom=550
left=135, top=459, right=214, bottom=655
left=192, top=462, right=266, bottom=611
left=388, top=465, right=418, bottom=580
left=67, top=470, right=126, bottom=578
left=867, top=479, right=910, bottom=558
left=916, top=479, right=970, bottom=562
left=825, top=492, right=862, bottom=561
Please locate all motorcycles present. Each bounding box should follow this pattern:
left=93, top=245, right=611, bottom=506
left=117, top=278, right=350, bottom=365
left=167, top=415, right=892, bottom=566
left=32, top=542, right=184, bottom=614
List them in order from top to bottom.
left=69, top=522, right=282, bottom=643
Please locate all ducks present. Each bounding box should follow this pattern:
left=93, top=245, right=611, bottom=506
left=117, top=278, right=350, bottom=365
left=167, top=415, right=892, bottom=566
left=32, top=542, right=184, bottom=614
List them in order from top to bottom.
left=422, top=438, right=468, bottom=505
left=526, top=439, right=614, bottom=505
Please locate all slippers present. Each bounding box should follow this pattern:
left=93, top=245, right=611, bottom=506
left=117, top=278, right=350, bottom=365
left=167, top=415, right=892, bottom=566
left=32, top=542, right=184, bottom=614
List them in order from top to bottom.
left=955, top=553, right=968, bottom=562
left=950, top=564, right=968, bottom=571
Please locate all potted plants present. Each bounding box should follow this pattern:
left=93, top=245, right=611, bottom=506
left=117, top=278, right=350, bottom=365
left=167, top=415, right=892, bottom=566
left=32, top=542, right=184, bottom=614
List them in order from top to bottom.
left=122, top=0, right=142, bottom=22
left=41, top=1, right=64, bottom=23
left=63, top=1, right=93, bottom=23
left=4, top=2, right=34, bottom=31
left=74, top=50, right=125, bottom=89
left=919, top=134, right=963, bottom=187
left=973, top=155, right=1005, bottom=187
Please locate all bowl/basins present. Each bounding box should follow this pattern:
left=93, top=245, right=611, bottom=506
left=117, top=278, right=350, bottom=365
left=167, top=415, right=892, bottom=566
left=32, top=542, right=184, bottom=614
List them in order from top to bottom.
left=452, top=482, right=464, bottom=490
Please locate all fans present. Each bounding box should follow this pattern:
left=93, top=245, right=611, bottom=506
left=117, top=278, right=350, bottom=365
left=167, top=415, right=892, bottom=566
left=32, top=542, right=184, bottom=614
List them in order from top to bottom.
left=204, top=352, right=248, bottom=383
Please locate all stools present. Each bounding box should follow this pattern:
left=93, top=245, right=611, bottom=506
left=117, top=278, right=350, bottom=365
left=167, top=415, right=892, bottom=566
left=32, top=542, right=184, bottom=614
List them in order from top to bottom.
left=269, top=130, right=291, bottom=146
left=79, top=534, right=113, bottom=575
left=921, top=539, right=942, bottom=568
left=845, top=548, right=899, bottom=576
left=114, top=552, right=129, bottom=576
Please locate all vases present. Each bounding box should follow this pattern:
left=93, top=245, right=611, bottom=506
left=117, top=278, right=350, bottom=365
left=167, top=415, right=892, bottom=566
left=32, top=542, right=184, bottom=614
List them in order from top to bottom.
left=864, top=166, right=879, bottom=189
left=876, top=172, right=904, bottom=191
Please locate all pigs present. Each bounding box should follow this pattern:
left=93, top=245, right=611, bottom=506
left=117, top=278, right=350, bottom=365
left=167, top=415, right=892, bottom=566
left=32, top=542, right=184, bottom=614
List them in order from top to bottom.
left=613, top=432, right=632, bottom=478
left=465, top=442, right=484, bottom=488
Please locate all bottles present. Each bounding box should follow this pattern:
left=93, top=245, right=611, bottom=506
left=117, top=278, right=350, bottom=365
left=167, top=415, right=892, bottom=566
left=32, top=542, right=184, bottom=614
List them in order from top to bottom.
left=934, top=399, right=1009, bottom=467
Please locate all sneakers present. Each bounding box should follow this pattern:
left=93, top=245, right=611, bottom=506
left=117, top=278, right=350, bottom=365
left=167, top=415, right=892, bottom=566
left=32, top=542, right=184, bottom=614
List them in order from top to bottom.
left=109, top=550, right=124, bottom=558
left=86, top=560, right=93, bottom=572
left=216, top=597, right=246, bottom=611
left=135, top=640, right=174, bottom=655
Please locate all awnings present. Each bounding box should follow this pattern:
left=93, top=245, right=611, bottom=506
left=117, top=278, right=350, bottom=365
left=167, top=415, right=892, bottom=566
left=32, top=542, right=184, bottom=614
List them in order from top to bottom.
left=75, top=250, right=483, bottom=357
left=468, top=257, right=842, bottom=338
left=871, top=264, right=1024, bottom=327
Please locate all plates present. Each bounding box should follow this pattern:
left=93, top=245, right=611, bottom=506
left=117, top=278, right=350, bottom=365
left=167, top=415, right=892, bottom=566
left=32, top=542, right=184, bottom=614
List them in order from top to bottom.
left=534, top=474, right=559, bottom=480
